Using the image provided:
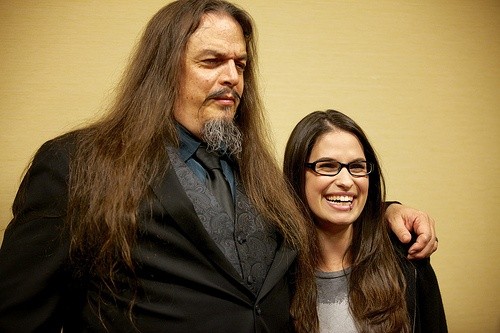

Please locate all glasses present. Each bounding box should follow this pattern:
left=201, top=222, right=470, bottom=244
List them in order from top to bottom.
left=304, top=159, right=374, bottom=177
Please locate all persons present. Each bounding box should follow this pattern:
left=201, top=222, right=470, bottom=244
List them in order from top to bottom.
left=0, top=1, right=439, bottom=332
left=278, top=108, right=450, bottom=332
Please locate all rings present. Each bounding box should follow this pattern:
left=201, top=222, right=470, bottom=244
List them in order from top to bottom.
left=435, top=237, right=439, bottom=243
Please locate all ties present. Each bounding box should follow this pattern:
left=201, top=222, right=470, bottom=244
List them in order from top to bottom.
left=194, top=148, right=236, bottom=221
